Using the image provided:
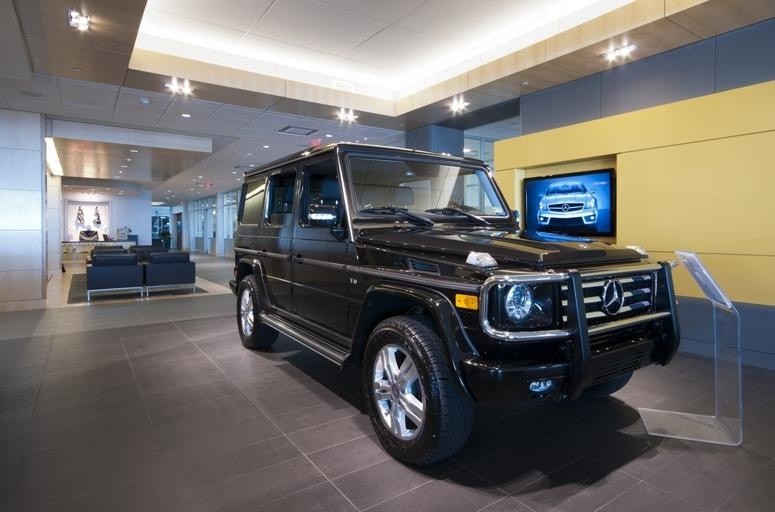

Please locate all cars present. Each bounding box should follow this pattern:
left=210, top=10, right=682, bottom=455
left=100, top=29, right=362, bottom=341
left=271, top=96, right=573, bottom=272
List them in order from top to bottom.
left=537, top=181, right=600, bottom=231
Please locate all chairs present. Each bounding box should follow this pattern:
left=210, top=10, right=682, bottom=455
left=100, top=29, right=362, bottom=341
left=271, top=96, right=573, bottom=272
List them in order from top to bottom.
left=282, top=179, right=416, bottom=226
left=85, top=245, right=197, bottom=301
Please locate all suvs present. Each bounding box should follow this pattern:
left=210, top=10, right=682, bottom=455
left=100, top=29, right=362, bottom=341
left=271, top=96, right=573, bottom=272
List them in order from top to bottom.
left=229, top=143, right=680, bottom=466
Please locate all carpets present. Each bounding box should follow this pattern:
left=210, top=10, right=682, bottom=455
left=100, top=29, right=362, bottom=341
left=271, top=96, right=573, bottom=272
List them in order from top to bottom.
left=67, top=272, right=210, bottom=304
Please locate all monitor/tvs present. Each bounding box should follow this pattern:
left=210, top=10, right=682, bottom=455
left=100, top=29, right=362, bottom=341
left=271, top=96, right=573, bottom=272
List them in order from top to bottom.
left=522, top=167, right=614, bottom=237
